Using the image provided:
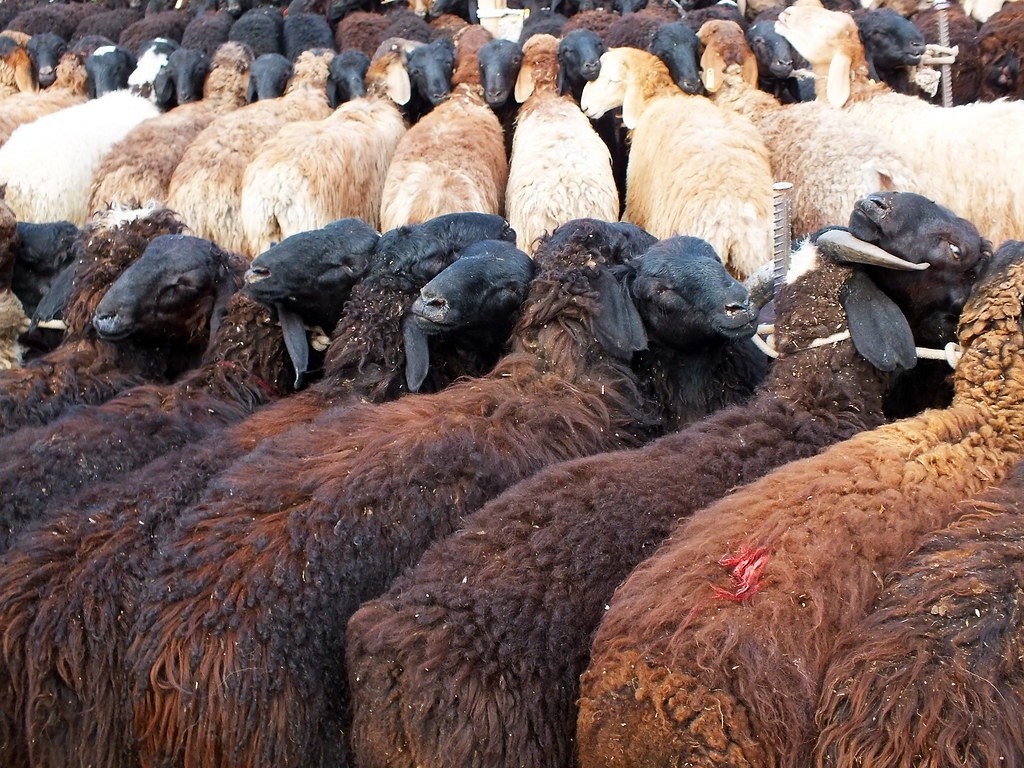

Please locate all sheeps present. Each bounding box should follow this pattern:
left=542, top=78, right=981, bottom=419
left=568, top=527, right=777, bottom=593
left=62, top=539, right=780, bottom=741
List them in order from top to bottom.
left=1, top=0, right=1022, bottom=765
